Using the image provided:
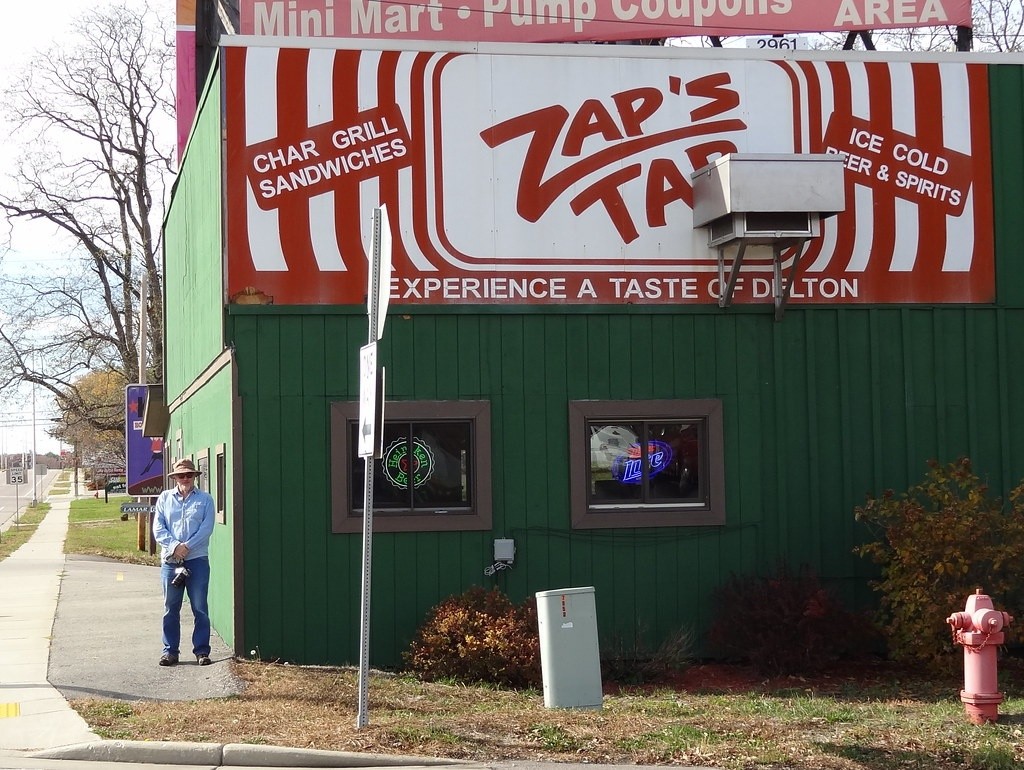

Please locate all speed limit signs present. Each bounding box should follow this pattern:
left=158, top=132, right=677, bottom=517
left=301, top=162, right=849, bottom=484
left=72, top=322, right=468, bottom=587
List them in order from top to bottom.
left=9, top=467, right=25, bottom=485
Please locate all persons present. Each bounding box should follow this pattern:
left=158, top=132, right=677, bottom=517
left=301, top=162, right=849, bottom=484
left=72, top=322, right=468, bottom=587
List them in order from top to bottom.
left=151, top=459, right=216, bottom=666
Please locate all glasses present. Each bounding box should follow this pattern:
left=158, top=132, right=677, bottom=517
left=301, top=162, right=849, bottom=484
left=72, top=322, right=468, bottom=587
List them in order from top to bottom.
left=176, top=472, right=195, bottom=480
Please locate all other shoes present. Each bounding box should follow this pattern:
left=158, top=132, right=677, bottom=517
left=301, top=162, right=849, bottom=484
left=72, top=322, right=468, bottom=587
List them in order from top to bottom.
left=159, top=653, right=179, bottom=665
left=196, top=653, right=212, bottom=666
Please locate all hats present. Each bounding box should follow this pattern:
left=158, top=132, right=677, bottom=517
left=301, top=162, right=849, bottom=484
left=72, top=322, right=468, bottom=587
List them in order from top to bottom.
left=167, top=459, right=203, bottom=479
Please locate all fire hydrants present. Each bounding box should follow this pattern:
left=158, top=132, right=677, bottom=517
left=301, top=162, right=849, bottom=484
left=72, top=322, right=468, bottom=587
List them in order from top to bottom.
left=945, top=587, right=1015, bottom=728
